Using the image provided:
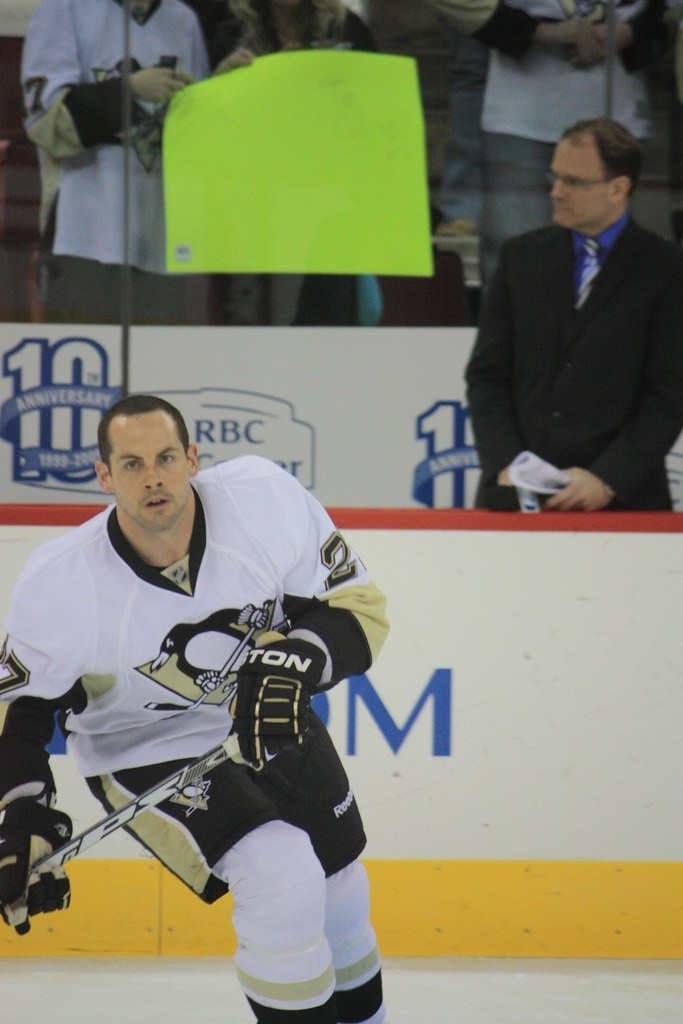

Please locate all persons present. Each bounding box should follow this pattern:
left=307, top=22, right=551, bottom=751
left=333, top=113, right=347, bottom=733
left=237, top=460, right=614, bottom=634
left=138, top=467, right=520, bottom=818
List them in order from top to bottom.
left=20, top=0, right=683, bottom=324
left=466, top=117, right=683, bottom=511
left=0, top=394, right=391, bottom=1024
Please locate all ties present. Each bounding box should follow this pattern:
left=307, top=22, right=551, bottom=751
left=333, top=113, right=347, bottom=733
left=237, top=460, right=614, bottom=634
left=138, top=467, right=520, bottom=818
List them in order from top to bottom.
left=575, top=238, right=600, bottom=309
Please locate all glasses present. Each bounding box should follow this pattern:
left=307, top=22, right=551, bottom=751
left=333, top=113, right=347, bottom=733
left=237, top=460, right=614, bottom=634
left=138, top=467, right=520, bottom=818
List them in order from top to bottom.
left=545, top=170, right=610, bottom=189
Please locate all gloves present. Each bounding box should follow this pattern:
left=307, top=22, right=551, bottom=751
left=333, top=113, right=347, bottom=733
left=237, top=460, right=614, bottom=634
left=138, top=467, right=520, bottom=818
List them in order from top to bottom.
left=227, top=637, right=328, bottom=771
left=0, top=800, right=72, bottom=936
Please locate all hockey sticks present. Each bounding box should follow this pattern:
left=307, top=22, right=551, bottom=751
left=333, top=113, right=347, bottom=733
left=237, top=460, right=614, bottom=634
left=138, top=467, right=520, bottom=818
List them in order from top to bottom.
left=24, top=736, right=242, bottom=887
